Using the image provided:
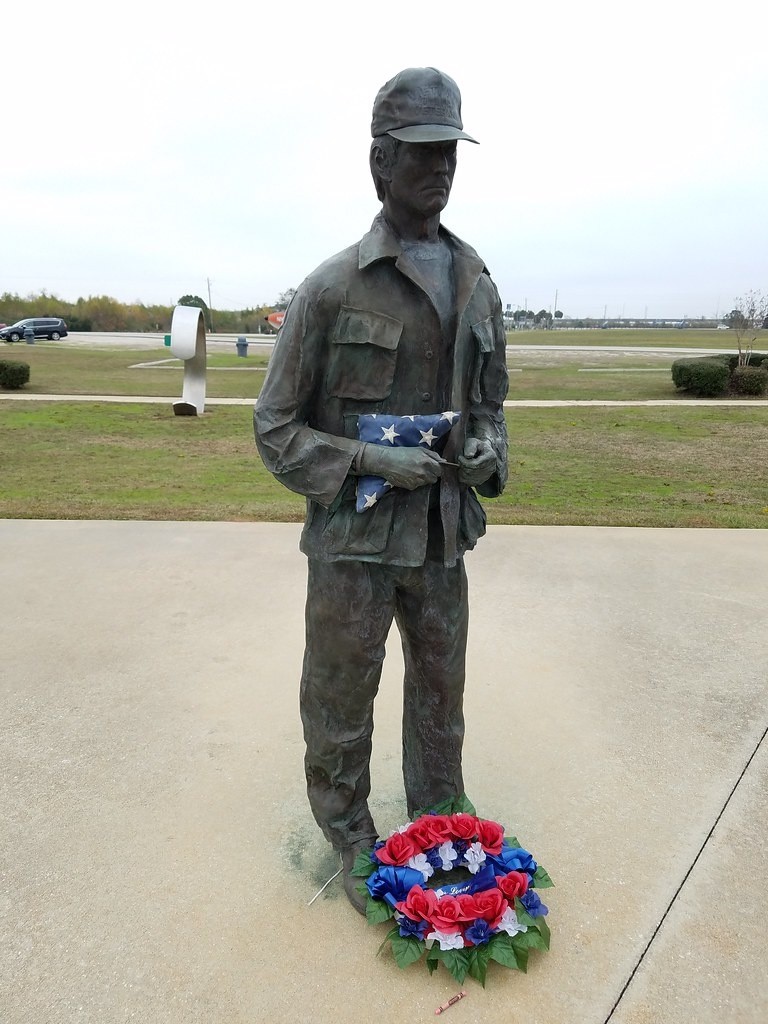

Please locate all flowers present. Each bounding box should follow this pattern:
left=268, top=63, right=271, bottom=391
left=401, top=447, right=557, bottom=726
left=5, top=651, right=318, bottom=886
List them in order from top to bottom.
left=352, top=790, right=556, bottom=991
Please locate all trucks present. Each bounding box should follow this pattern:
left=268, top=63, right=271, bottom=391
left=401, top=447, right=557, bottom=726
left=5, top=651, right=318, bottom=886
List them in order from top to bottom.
left=717, top=324, right=729, bottom=330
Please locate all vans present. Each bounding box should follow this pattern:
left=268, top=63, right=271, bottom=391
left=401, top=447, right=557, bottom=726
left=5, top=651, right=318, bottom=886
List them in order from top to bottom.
left=0, top=318, right=68, bottom=342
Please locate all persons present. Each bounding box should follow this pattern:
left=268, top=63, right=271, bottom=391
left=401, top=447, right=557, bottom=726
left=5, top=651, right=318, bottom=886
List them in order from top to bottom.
left=252, top=66, right=508, bottom=918
